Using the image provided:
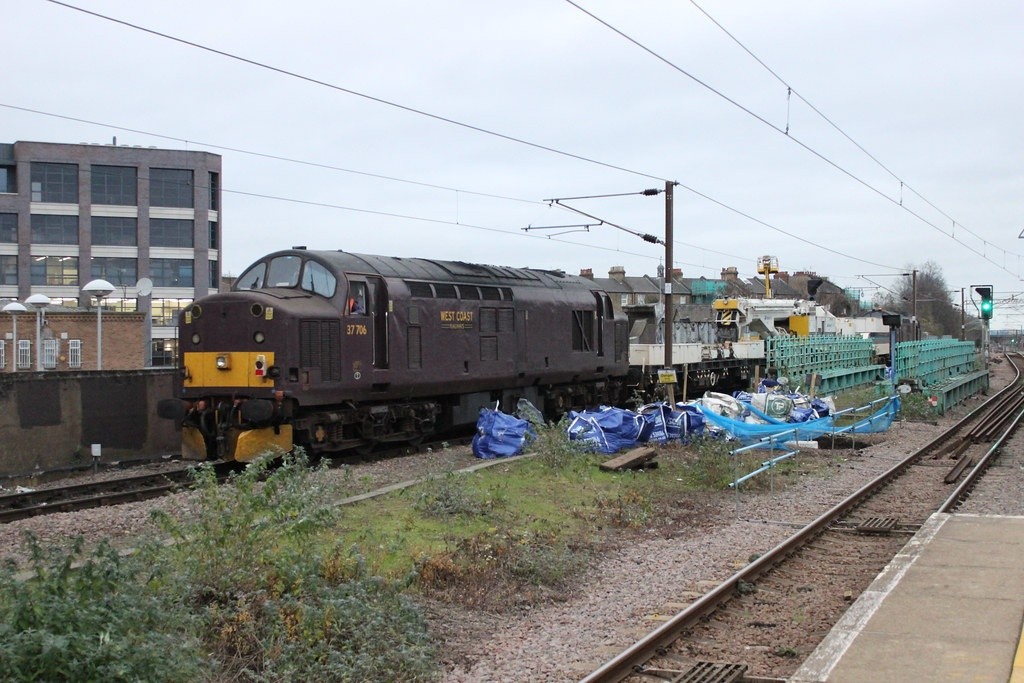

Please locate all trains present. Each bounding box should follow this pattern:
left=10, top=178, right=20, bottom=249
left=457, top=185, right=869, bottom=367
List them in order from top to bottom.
left=159, top=247, right=921, bottom=463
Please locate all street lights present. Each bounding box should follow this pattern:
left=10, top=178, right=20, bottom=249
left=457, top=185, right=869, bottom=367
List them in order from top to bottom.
left=82, top=279, right=117, bottom=369
left=24, top=294, right=50, bottom=371
left=2, top=302, right=27, bottom=372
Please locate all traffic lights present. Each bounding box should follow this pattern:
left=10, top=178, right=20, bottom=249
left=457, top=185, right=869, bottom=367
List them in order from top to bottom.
left=807, top=279, right=823, bottom=295
left=976, top=287, right=991, bottom=318
